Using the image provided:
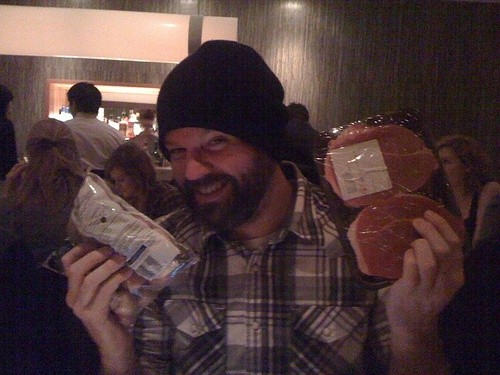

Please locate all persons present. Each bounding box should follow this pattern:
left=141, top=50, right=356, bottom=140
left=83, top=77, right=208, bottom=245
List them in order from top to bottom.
left=60, top=39, right=465, bottom=375
left=62, top=81, right=126, bottom=170
left=0, top=83, right=18, bottom=180
left=286, top=103, right=327, bottom=187
left=128, top=109, right=168, bottom=162
left=1, top=117, right=128, bottom=375
left=435, top=133, right=500, bottom=253
left=103, top=142, right=186, bottom=218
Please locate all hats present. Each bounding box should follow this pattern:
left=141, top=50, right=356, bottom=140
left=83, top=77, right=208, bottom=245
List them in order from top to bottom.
left=156, top=40, right=284, bottom=162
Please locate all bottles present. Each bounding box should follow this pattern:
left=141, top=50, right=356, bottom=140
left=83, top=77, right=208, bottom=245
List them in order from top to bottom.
left=53, top=107, right=158, bottom=140
